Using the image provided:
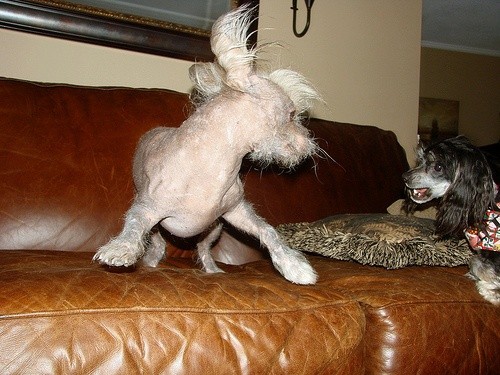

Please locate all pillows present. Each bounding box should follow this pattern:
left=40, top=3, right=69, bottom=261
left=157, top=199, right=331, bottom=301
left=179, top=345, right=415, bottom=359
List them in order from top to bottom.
left=275, top=198, right=475, bottom=270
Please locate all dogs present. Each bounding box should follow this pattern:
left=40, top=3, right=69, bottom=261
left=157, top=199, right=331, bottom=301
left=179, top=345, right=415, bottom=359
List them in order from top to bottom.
left=92, top=0, right=345, bottom=286
left=402, top=134, right=500, bottom=306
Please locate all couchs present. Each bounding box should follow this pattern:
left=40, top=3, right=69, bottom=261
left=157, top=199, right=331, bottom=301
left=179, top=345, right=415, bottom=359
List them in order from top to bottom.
left=0, top=78, right=500, bottom=375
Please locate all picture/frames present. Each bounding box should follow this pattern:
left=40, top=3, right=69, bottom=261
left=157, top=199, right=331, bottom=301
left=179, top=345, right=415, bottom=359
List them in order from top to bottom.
left=0, top=0, right=259, bottom=69
